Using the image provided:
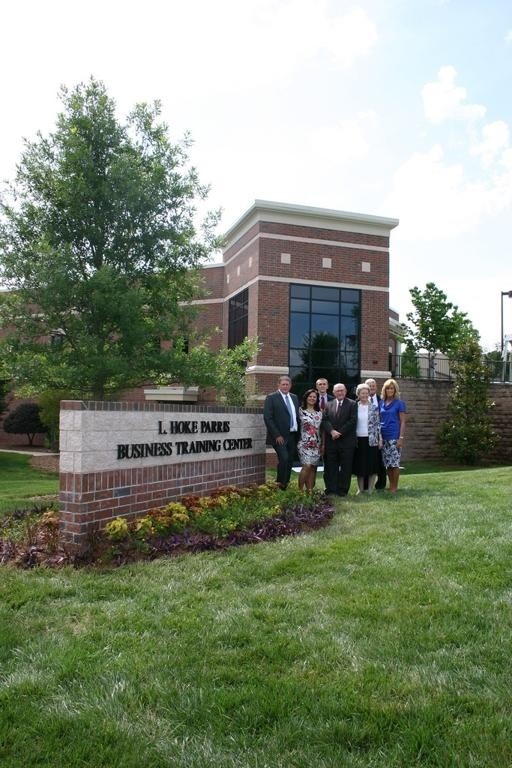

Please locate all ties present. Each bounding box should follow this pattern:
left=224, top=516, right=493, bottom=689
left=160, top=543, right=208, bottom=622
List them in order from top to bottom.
left=370, top=394, right=373, bottom=403
left=285, top=396, right=293, bottom=428
left=321, top=397, right=325, bottom=411
left=336, top=399, right=341, bottom=420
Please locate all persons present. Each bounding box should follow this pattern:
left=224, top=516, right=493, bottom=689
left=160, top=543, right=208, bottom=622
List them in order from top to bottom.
left=263, top=375, right=407, bottom=498
